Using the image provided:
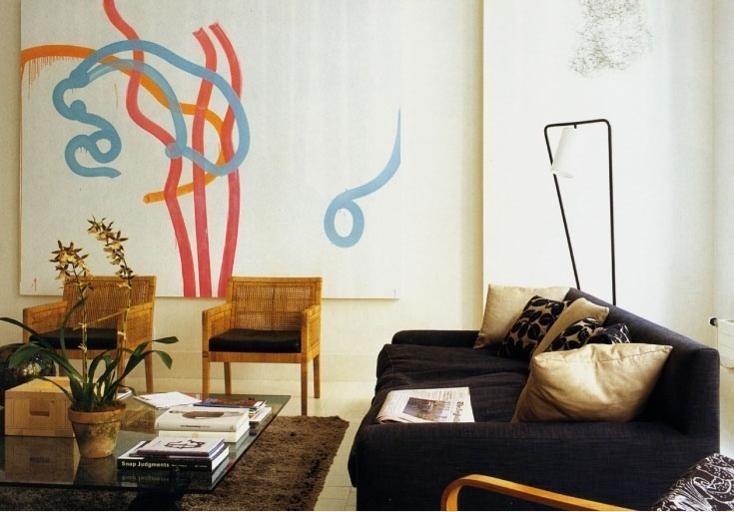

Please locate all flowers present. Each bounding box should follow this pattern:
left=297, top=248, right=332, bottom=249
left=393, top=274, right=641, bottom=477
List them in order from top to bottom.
left=3, top=220, right=179, bottom=412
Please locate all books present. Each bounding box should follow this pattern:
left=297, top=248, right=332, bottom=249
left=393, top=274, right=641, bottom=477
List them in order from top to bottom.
left=375, top=385, right=476, bottom=425
left=132, top=391, right=202, bottom=408
left=116, top=393, right=272, bottom=472
left=115, top=466, right=220, bottom=488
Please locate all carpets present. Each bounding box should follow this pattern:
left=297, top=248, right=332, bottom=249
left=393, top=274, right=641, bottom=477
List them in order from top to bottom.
left=1, top=415, right=349, bottom=511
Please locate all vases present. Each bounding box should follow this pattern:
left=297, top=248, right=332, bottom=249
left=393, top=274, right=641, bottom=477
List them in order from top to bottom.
left=69, top=402, right=128, bottom=458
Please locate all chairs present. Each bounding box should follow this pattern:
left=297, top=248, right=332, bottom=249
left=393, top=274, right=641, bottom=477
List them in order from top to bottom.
left=23, top=275, right=157, bottom=389
left=203, top=277, right=322, bottom=415
left=440, top=473, right=633, bottom=511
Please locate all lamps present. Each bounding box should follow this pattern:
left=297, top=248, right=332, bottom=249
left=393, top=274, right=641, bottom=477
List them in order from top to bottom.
left=542, top=116, right=617, bottom=303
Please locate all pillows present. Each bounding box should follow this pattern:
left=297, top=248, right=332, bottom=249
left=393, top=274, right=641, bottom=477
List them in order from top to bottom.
left=474, top=282, right=673, bottom=423
left=651, top=452, right=734, bottom=512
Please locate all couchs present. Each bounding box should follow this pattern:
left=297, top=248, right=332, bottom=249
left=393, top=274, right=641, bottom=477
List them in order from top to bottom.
left=350, top=288, right=720, bottom=510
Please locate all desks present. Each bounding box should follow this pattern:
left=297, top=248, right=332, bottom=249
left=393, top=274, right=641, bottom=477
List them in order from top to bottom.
left=0, top=393, right=291, bottom=492
